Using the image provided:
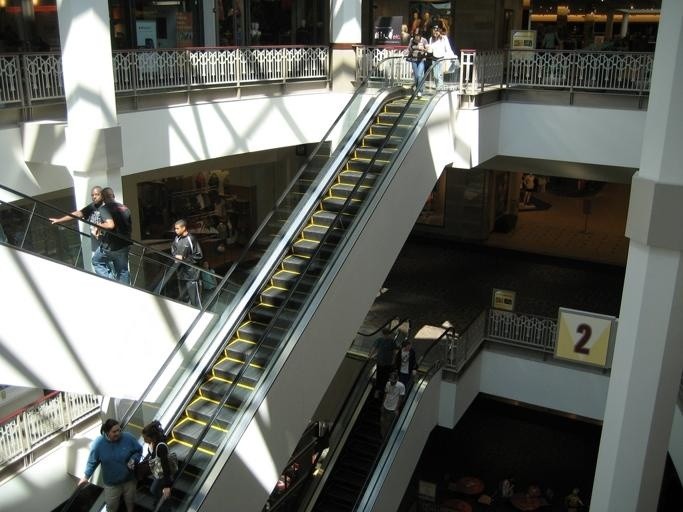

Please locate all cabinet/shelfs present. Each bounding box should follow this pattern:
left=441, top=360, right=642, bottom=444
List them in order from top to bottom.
left=222, top=181, right=257, bottom=248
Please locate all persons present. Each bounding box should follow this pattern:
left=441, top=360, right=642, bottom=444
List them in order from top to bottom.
left=410, top=12, right=443, bottom=43
left=541, top=24, right=561, bottom=50
left=425, top=25, right=458, bottom=94
left=402, top=28, right=430, bottom=97
left=523, top=172, right=536, bottom=206
left=49, top=186, right=120, bottom=282
left=92, top=187, right=132, bottom=285
left=170, top=219, right=204, bottom=309
left=199, top=261, right=217, bottom=302
left=369, top=327, right=419, bottom=441
left=77, top=419, right=143, bottom=512
left=130, top=420, right=172, bottom=501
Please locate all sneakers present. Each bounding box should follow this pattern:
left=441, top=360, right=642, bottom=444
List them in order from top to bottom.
left=414, top=91, right=422, bottom=99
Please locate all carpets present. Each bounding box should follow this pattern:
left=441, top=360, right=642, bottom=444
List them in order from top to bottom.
left=518, top=191, right=551, bottom=211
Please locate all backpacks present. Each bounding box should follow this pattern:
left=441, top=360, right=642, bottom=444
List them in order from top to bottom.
left=89, top=211, right=110, bottom=241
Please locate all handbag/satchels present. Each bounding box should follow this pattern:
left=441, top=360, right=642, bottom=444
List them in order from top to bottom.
left=406, top=51, right=422, bottom=63
left=442, top=55, right=457, bottom=75
left=147, top=442, right=180, bottom=480
